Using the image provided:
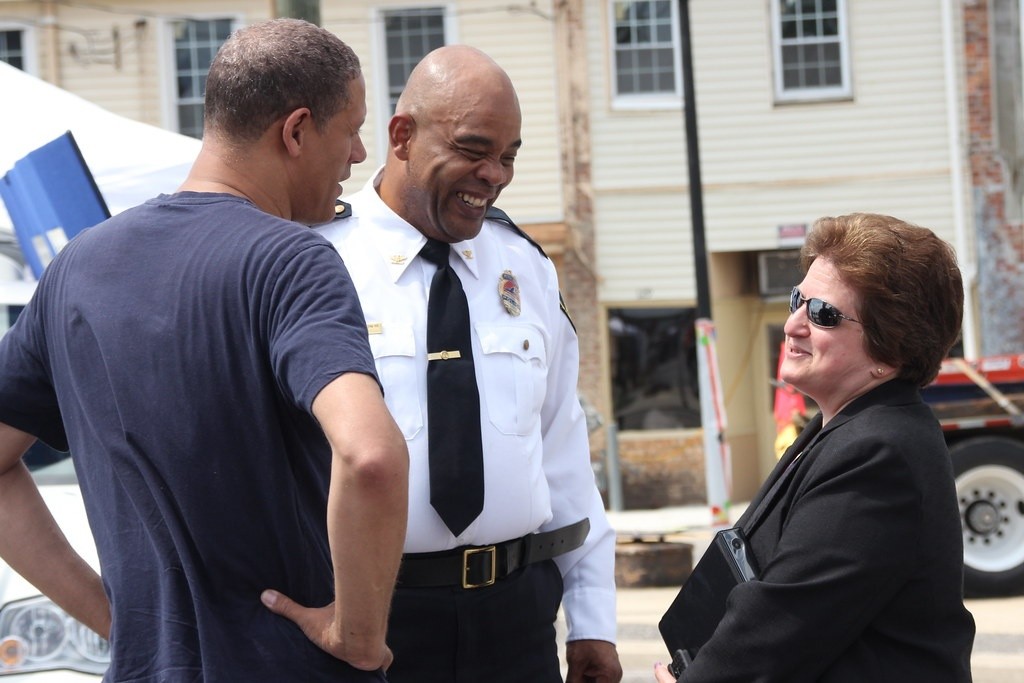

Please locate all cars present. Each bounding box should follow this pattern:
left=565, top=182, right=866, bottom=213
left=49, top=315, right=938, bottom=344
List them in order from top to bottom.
left=1, top=278, right=110, bottom=683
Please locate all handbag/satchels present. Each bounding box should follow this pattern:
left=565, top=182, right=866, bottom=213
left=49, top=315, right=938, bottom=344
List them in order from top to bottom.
left=657, top=526, right=762, bottom=677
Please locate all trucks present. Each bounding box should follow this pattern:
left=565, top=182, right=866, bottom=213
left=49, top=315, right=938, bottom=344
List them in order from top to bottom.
left=915, top=357, right=1024, bottom=601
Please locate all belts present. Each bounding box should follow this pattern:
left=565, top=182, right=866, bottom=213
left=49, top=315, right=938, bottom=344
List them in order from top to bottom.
left=396, top=517, right=590, bottom=589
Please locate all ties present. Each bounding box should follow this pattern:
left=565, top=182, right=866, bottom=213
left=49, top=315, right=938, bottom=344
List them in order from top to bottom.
left=419, top=238, right=484, bottom=538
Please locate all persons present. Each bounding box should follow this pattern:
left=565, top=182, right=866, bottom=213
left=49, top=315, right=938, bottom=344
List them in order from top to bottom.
left=0, top=16, right=411, bottom=683
left=308, top=43, right=624, bottom=683
left=656, top=214, right=976, bottom=683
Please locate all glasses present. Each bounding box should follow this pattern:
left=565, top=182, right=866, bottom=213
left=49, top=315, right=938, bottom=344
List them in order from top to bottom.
left=788, top=286, right=876, bottom=332
